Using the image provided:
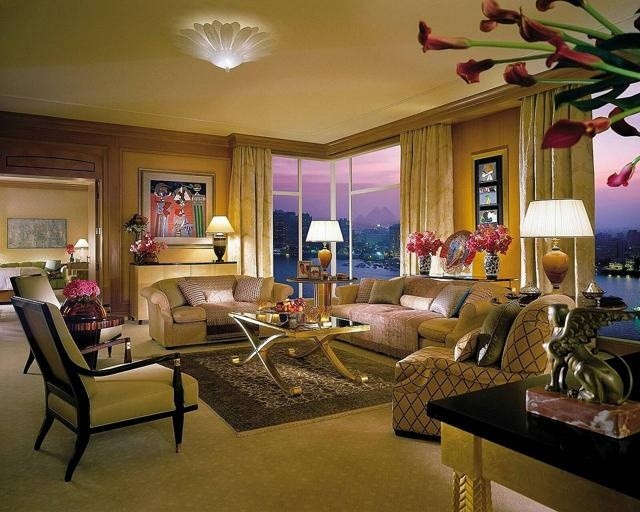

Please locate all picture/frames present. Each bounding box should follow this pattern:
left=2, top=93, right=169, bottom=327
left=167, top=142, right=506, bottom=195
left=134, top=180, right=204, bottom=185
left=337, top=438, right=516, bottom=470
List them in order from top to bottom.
left=471, top=147, right=508, bottom=231
left=138, top=167, right=217, bottom=249
left=6, top=218, right=67, bottom=249
left=296, top=260, right=321, bottom=280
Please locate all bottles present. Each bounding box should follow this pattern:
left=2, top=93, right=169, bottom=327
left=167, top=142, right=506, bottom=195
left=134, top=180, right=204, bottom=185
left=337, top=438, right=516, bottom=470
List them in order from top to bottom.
left=321, top=269, right=329, bottom=281
left=582, top=281, right=605, bottom=299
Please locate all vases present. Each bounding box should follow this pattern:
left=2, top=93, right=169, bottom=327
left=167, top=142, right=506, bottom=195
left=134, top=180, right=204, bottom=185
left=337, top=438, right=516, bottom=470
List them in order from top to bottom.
left=133, top=233, right=144, bottom=265
left=484, top=251, right=500, bottom=280
left=142, top=252, right=159, bottom=264
left=70, top=253, right=75, bottom=262
left=420, top=254, right=432, bottom=275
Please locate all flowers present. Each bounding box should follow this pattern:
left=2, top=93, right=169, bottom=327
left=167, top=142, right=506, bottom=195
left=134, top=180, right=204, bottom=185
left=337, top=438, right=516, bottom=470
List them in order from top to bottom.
left=417, top=0, right=640, bottom=188
left=124, top=214, right=148, bottom=234
left=465, top=223, right=513, bottom=255
left=407, top=230, right=443, bottom=256
left=130, top=233, right=168, bottom=254
left=65, top=244, right=75, bottom=254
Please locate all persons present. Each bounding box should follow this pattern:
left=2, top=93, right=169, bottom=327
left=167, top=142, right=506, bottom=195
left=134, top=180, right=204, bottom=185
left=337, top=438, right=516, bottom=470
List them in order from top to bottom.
left=300, top=263, right=308, bottom=276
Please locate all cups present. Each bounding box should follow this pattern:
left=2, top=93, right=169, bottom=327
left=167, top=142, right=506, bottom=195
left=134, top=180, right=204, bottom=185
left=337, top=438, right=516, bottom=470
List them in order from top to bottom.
left=256, top=296, right=333, bottom=329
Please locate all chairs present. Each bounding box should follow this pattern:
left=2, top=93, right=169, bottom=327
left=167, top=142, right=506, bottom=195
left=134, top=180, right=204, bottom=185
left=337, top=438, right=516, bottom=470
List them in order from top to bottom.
left=9, top=275, right=198, bottom=483
left=393, top=294, right=575, bottom=436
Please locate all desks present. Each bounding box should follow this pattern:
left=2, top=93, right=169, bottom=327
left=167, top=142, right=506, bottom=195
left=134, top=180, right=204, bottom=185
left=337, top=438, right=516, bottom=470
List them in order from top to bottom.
left=67, top=261, right=88, bottom=280
left=427, top=356, right=640, bottom=512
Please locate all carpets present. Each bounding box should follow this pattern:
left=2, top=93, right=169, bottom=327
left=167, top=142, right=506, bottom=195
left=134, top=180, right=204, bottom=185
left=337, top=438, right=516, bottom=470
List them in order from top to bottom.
left=160, top=339, right=394, bottom=438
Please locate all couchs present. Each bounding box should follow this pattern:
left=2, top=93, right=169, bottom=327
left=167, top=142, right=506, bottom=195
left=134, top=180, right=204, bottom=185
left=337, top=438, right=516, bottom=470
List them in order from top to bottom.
left=0, top=262, right=66, bottom=288
left=139, top=275, right=295, bottom=348
left=330, top=275, right=508, bottom=358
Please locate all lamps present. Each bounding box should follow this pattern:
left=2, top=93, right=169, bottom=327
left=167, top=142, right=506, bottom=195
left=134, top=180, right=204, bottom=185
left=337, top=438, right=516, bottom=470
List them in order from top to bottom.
left=172, top=18, right=278, bottom=74
left=519, top=198, right=595, bottom=296
left=205, top=215, right=234, bottom=262
left=305, top=220, right=344, bottom=277
left=74, top=239, right=88, bottom=260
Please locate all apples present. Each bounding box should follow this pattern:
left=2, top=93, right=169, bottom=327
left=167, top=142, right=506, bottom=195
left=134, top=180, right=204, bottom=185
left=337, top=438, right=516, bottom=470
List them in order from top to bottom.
left=276, top=299, right=303, bottom=312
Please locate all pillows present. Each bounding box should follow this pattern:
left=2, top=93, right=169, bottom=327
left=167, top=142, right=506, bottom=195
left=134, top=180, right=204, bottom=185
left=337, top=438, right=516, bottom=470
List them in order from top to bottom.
left=45, top=259, right=61, bottom=270
left=356, top=276, right=470, bottom=317
left=178, top=277, right=263, bottom=306
left=454, top=300, right=519, bottom=367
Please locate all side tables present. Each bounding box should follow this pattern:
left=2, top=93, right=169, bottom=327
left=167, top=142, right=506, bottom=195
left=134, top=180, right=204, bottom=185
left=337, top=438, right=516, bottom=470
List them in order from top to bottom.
left=285, top=277, right=352, bottom=306
left=489, top=289, right=626, bottom=311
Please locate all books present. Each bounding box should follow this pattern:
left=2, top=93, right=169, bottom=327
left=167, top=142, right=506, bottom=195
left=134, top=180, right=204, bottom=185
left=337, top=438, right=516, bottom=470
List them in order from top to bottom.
left=336, top=272, right=348, bottom=281
